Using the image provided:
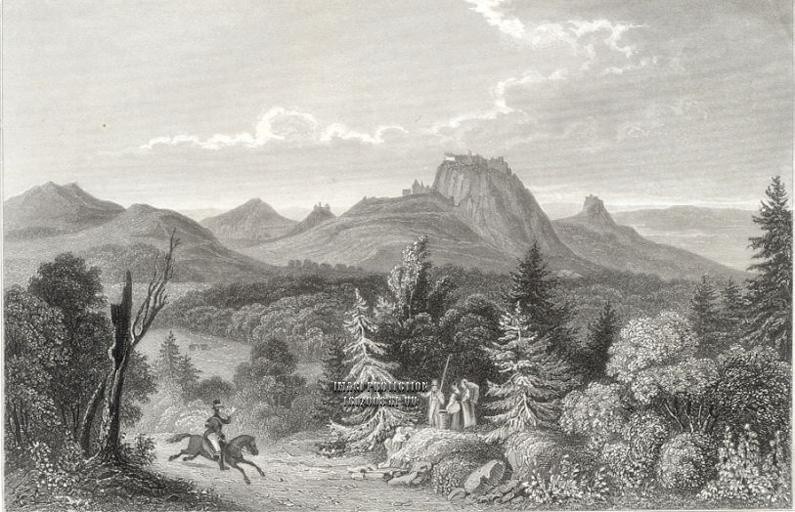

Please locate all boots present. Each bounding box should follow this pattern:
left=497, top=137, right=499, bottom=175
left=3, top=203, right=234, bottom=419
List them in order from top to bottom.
left=217, top=454, right=230, bottom=470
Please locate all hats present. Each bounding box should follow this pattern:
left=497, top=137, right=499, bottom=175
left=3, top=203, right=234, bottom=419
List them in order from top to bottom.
left=213, top=400, right=223, bottom=407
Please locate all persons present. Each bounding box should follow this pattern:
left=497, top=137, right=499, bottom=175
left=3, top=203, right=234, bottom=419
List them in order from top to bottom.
left=459, top=377, right=478, bottom=432
left=419, top=379, right=446, bottom=429
left=447, top=379, right=463, bottom=431
left=204, top=399, right=235, bottom=472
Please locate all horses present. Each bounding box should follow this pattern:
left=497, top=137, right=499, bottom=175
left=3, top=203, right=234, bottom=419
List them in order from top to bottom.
left=165, top=432, right=265, bottom=486
